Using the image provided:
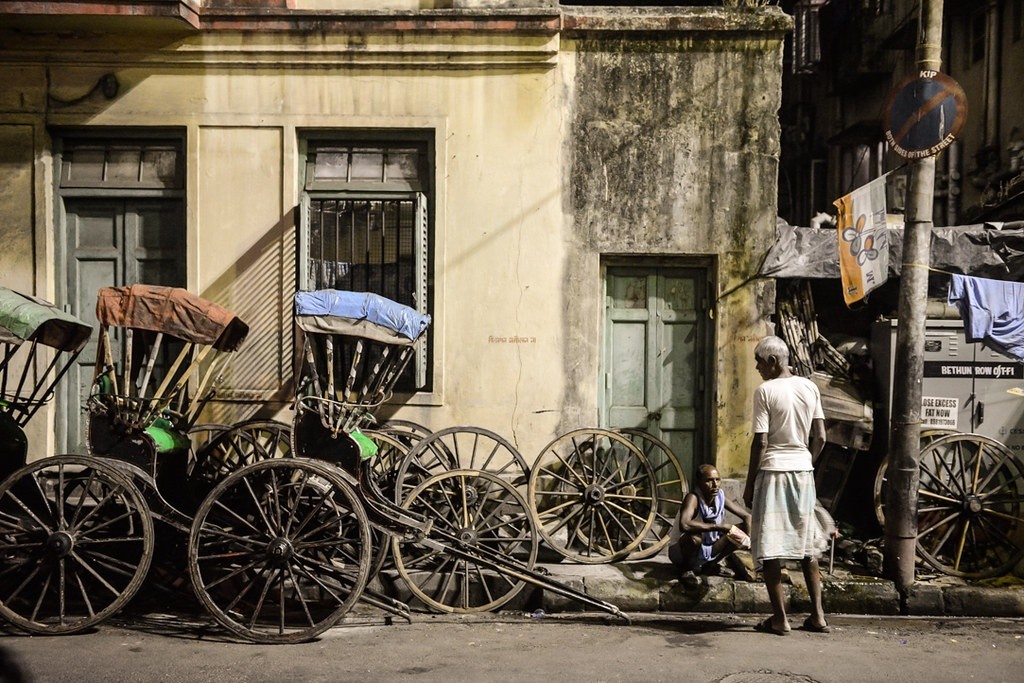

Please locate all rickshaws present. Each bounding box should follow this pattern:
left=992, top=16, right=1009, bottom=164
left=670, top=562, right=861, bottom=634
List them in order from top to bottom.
left=0, top=281, right=1023, bottom=647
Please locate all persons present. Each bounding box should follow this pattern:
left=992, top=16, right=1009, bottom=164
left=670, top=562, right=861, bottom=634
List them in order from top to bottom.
left=744, top=336, right=836, bottom=635
left=668, top=463, right=755, bottom=592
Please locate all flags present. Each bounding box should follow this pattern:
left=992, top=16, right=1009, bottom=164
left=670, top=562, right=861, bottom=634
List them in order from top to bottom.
left=833, top=174, right=895, bottom=309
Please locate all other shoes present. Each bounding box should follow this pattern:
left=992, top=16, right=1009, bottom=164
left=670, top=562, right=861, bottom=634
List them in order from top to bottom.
left=681, top=570, right=699, bottom=584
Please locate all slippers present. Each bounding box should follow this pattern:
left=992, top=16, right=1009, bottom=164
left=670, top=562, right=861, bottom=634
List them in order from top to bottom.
left=799, top=616, right=831, bottom=633
left=754, top=618, right=789, bottom=636
